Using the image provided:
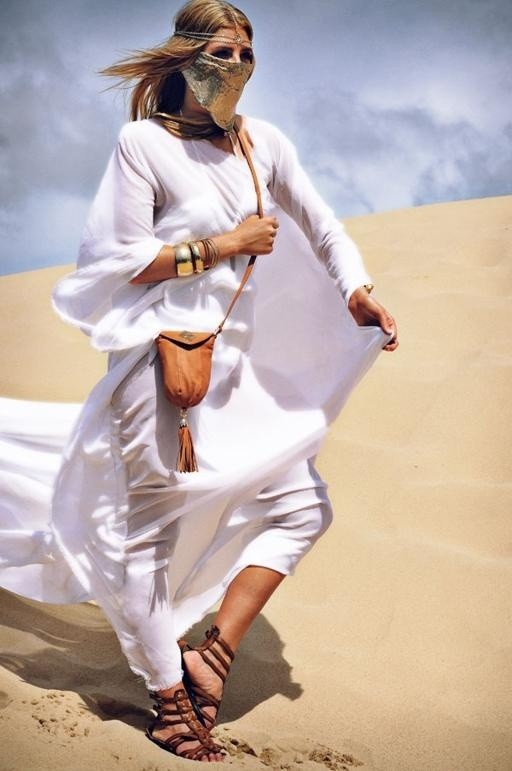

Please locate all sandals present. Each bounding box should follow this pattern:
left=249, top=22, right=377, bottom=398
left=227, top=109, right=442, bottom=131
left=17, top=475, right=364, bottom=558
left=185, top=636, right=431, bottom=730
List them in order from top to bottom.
left=145, top=624, right=235, bottom=761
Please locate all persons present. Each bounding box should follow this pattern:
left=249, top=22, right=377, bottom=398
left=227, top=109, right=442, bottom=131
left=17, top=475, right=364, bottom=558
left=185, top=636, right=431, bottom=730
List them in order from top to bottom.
left=0, top=0, right=398, bottom=764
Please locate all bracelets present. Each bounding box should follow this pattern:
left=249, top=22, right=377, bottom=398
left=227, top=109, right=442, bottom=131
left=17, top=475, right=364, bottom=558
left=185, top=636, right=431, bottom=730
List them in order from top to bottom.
left=174, top=238, right=220, bottom=277
left=363, top=283, right=376, bottom=296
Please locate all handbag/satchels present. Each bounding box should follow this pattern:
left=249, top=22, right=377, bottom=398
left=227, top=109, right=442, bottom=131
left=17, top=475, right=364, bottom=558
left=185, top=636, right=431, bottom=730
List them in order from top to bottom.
left=156, top=330, right=216, bottom=471
